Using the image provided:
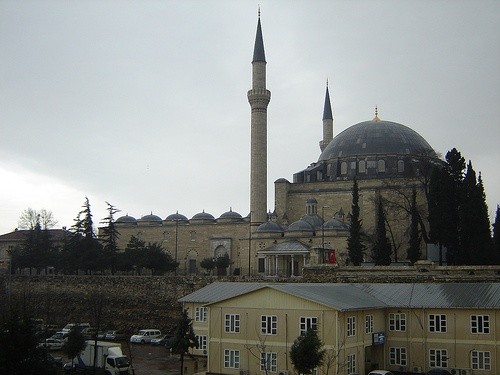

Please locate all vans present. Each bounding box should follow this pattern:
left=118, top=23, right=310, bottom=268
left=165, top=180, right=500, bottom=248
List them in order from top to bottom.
left=62, top=324, right=90, bottom=335
left=81, top=328, right=101, bottom=338
left=130, top=329, right=161, bottom=345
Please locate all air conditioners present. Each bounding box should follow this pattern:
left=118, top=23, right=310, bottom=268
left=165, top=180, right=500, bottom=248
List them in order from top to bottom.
left=201, top=349, right=209, bottom=356
left=412, top=367, right=422, bottom=373
left=449, top=368, right=460, bottom=375
left=461, top=368, right=472, bottom=375
left=278, top=369, right=290, bottom=375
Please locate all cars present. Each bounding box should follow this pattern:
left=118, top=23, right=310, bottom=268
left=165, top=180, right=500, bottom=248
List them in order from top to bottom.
left=105, top=331, right=130, bottom=342
left=96, top=332, right=109, bottom=340
left=151, top=333, right=174, bottom=346
left=38, top=338, right=57, bottom=349
left=368, top=370, right=394, bottom=375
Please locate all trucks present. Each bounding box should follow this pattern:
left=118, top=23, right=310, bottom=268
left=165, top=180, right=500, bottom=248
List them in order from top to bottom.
left=54, top=332, right=68, bottom=347
left=74, top=340, right=131, bottom=375
